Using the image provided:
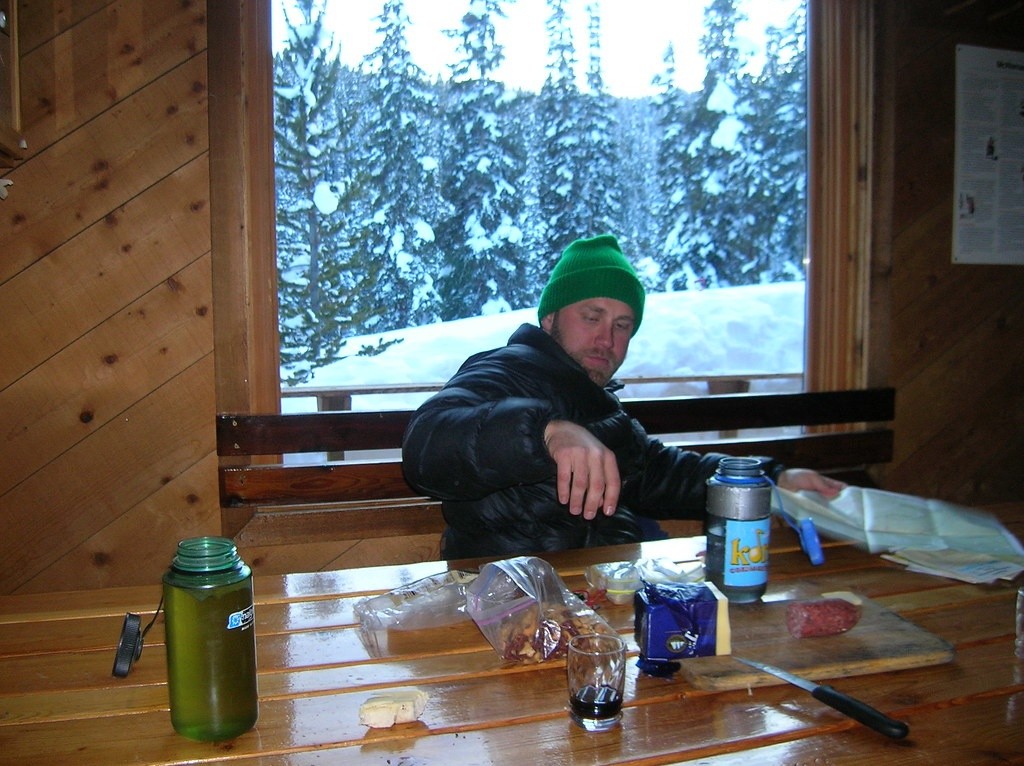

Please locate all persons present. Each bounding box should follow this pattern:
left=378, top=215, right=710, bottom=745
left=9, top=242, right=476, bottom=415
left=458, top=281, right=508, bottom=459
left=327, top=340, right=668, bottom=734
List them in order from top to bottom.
left=404, top=232, right=842, bottom=558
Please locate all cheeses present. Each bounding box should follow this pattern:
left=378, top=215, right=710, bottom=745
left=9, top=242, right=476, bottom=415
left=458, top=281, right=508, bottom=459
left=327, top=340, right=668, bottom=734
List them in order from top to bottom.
left=633, top=581, right=732, bottom=659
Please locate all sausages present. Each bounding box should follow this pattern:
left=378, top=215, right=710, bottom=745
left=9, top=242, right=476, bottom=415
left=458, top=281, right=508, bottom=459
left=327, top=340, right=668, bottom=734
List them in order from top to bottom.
left=785, top=598, right=862, bottom=639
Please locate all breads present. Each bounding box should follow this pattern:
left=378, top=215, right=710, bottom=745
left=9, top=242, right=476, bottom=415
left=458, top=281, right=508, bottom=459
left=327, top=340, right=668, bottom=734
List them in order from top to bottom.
left=358, top=689, right=428, bottom=729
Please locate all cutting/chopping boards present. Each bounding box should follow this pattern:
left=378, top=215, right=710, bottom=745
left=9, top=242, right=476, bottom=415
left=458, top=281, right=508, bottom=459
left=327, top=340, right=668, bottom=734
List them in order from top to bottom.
left=670, top=593, right=955, bottom=692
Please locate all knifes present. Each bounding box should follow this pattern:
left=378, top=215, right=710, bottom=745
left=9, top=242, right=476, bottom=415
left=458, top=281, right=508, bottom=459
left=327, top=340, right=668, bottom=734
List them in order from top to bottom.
left=732, top=656, right=909, bottom=741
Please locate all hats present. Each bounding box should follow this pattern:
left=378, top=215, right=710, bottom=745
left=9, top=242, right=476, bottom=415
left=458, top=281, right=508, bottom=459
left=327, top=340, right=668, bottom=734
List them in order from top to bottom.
left=539, top=233, right=645, bottom=339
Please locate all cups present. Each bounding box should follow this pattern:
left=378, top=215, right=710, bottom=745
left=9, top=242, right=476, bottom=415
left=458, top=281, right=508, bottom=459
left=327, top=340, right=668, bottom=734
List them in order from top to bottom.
left=706, top=457, right=823, bottom=605
left=112, top=536, right=258, bottom=739
left=1014, top=587, right=1024, bottom=660
left=567, top=634, right=627, bottom=731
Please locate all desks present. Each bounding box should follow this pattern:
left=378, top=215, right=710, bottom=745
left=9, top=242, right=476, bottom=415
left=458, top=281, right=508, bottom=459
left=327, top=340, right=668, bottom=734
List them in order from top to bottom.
left=0, top=498, right=1024, bottom=766
